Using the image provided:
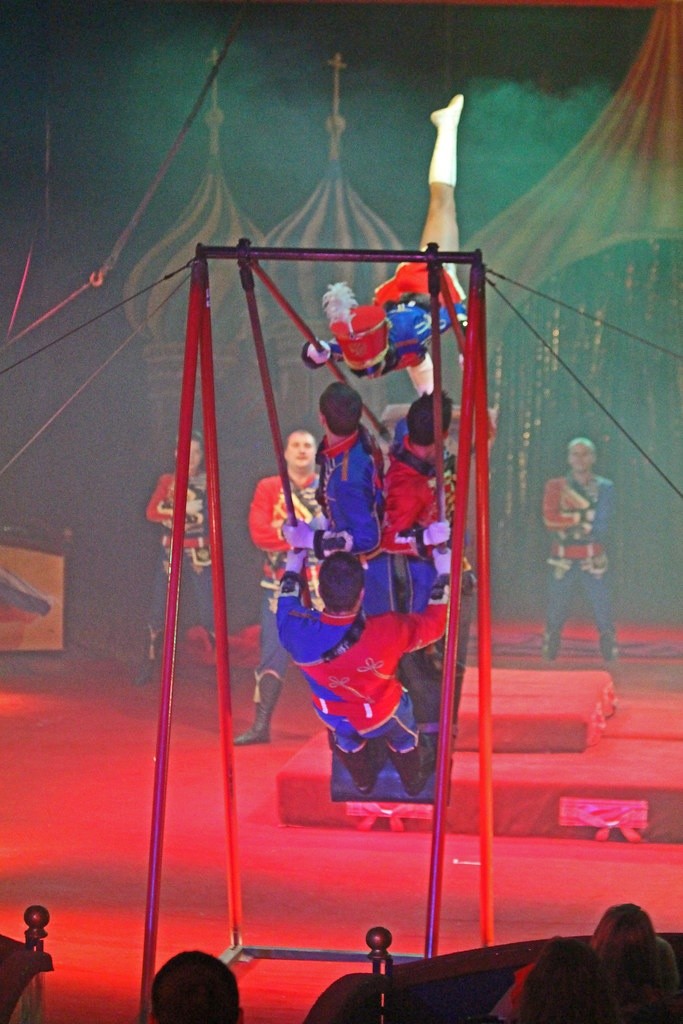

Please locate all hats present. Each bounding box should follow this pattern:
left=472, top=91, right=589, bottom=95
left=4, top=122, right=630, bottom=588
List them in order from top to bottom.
left=322, top=281, right=390, bottom=378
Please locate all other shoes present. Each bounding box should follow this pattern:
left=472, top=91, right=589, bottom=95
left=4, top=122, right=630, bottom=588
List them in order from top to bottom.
left=421, top=643, right=464, bottom=678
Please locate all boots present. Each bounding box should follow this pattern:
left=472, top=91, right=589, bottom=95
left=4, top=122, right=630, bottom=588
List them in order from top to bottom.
left=132, top=620, right=164, bottom=685
left=198, top=630, right=232, bottom=687
left=232, top=668, right=284, bottom=745
left=388, top=727, right=436, bottom=796
left=327, top=731, right=386, bottom=795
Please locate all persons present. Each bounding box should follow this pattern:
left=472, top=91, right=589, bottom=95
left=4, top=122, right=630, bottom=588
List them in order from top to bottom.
left=301, top=91, right=465, bottom=397
left=281, top=382, right=476, bottom=771
left=233, top=429, right=321, bottom=744
left=133, top=431, right=215, bottom=687
left=542, top=438, right=620, bottom=661
left=276, top=547, right=452, bottom=796
left=509, top=902, right=683, bottom=1024
left=149, top=951, right=244, bottom=1024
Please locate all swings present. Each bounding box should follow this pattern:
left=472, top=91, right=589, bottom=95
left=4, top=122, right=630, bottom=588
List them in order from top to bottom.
left=236, top=236, right=476, bottom=807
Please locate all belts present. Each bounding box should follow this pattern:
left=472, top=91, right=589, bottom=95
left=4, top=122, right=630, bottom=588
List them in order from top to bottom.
left=161, top=536, right=213, bottom=549
left=550, top=545, right=603, bottom=559
